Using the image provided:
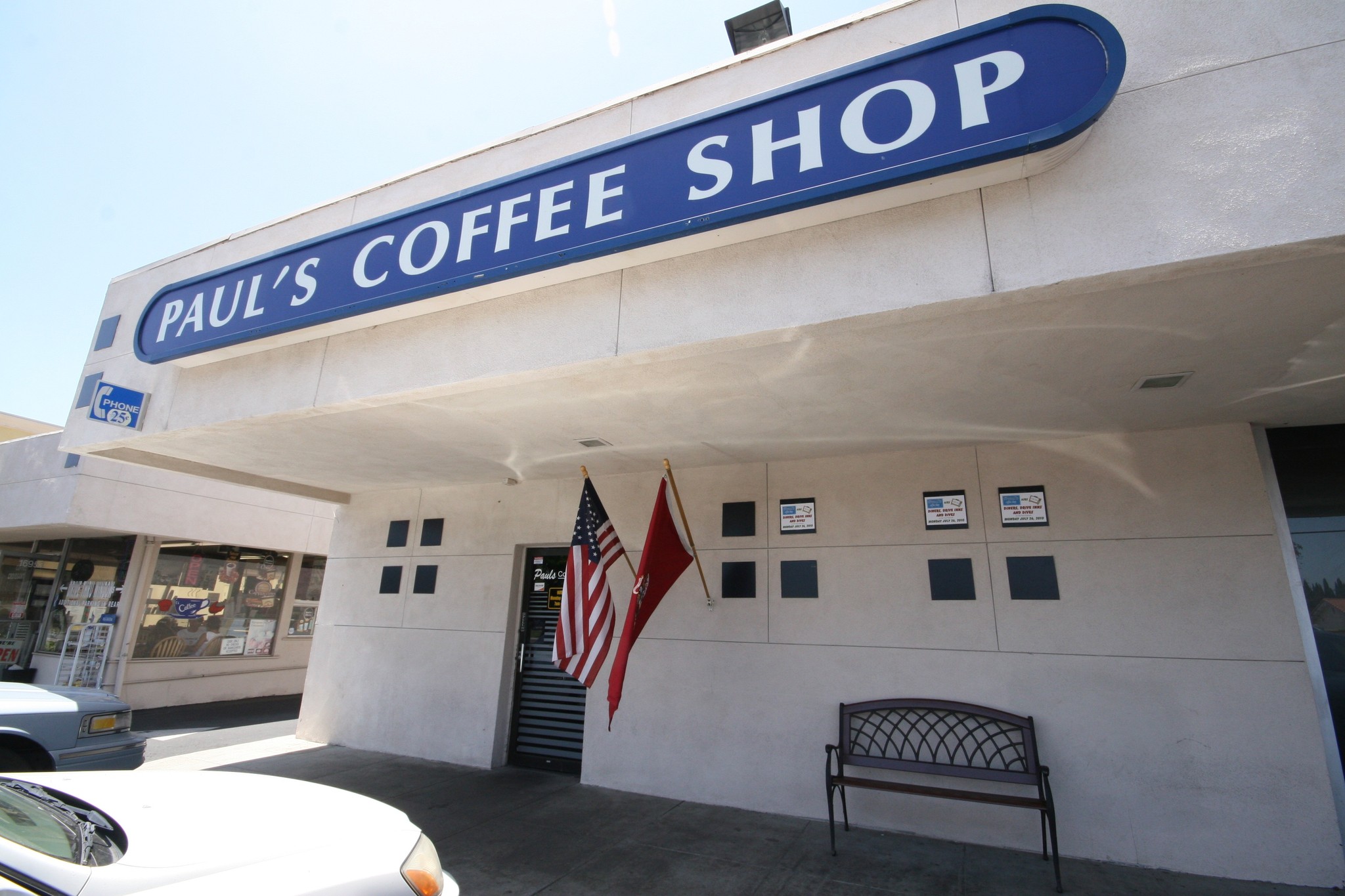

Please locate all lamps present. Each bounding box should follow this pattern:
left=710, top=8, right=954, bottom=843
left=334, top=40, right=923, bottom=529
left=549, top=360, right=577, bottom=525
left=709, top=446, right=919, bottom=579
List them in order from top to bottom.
left=723, top=0, right=793, bottom=56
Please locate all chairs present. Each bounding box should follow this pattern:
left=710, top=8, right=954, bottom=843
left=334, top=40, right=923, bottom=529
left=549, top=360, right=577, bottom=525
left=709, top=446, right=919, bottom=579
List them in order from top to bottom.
left=199, top=635, right=225, bottom=657
left=151, top=636, right=186, bottom=657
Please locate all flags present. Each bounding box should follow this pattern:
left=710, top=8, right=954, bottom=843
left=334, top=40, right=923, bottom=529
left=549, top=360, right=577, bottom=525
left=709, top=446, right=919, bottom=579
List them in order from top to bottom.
left=607, top=474, right=694, bottom=733
left=551, top=477, right=626, bottom=689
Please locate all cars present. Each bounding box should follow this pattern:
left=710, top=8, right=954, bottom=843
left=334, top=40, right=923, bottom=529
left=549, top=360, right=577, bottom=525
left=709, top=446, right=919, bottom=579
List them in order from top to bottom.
left=0, top=680, right=146, bottom=771
left=0, top=769, right=460, bottom=896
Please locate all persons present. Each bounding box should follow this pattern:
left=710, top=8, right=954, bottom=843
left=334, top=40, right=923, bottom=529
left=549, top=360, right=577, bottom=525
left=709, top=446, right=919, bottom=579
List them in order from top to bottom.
left=176, top=616, right=221, bottom=656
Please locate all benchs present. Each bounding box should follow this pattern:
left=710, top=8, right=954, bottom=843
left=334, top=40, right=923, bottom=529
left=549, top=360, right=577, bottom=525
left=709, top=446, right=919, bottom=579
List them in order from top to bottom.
left=823, top=700, right=1061, bottom=896
